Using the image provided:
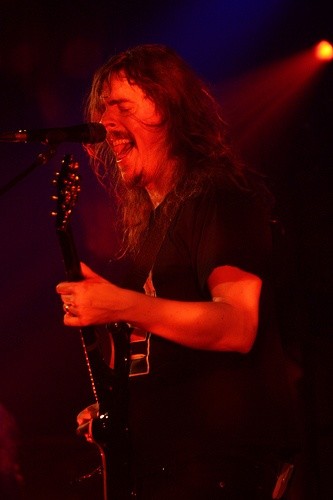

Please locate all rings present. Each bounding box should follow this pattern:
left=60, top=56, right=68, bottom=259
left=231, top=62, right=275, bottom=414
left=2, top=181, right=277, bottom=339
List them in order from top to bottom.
left=66, top=305, right=69, bottom=312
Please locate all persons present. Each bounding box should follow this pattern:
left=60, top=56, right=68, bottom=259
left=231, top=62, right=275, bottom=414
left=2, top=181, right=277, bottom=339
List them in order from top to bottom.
left=54, top=44, right=310, bottom=500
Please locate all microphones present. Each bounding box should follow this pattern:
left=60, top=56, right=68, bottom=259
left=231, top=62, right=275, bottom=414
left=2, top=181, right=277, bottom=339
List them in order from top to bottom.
left=0, top=122, right=107, bottom=144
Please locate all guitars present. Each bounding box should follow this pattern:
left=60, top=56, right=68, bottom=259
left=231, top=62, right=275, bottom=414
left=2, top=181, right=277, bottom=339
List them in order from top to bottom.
left=48, top=151, right=133, bottom=500
